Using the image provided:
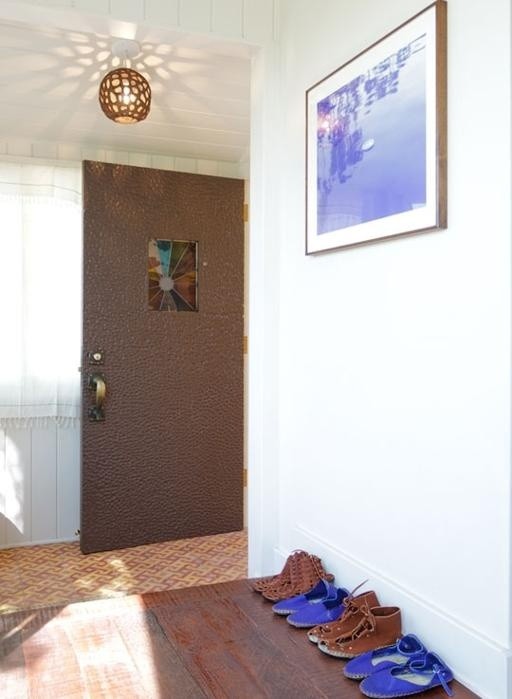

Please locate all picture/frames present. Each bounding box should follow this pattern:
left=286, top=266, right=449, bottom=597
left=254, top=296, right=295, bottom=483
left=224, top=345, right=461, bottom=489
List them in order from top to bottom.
left=304, top=0, right=448, bottom=259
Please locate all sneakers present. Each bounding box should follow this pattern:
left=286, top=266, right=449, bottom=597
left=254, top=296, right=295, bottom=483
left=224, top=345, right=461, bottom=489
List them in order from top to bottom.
left=271, top=578, right=453, bottom=699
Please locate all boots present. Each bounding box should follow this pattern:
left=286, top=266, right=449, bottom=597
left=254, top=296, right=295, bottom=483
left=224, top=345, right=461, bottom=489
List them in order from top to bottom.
left=253, top=551, right=307, bottom=593
left=263, top=555, right=334, bottom=604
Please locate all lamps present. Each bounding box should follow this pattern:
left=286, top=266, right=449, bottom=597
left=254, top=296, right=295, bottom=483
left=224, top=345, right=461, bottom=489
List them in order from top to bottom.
left=99, top=38, right=152, bottom=125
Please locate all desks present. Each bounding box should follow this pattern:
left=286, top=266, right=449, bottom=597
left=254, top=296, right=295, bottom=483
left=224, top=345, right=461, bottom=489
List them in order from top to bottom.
left=0, top=571, right=481, bottom=698
